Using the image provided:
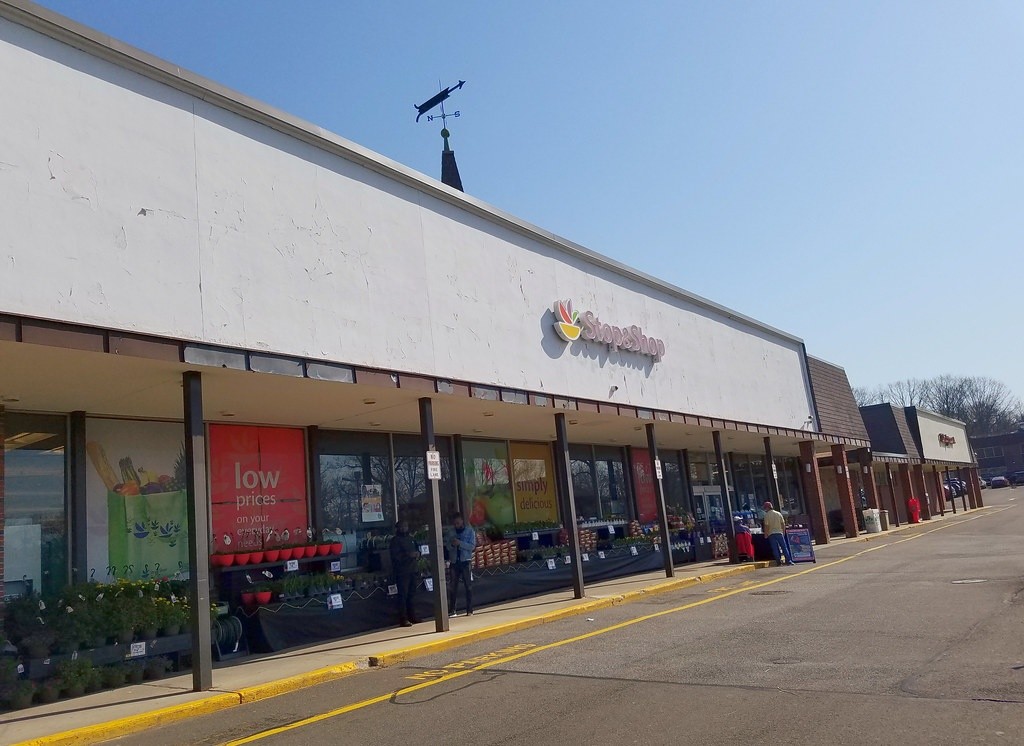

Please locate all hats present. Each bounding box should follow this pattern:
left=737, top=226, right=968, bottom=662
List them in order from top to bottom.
left=763, top=502, right=773, bottom=512
left=733, top=516, right=743, bottom=522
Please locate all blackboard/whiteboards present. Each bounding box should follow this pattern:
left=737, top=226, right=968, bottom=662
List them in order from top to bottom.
left=786, top=529, right=816, bottom=562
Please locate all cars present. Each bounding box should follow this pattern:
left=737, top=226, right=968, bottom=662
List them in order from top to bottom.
left=942, top=475, right=987, bottom=500
left=990, top=476, right=1010, bottom=489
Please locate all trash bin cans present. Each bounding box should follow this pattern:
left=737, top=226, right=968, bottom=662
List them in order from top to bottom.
left=862, top=508, right=889, bottom=533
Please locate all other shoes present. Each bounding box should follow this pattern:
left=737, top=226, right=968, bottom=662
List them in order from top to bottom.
left=787, top=560, right=795, bottom=566
left=777, top=563, right=783, bottom=567
left=467, top=611, right=473, bottom=615
left=449, top=613, right=457, bottom=617
left=409, top=619, right=423, bottom=623
left=400, top=620, right=412, bottom=627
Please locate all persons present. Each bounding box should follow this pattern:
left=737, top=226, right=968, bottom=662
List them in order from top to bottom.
left=763, top=502, right=796, bottom=566
left=445, top=512, right=477, bottom=618
left=734, top=516, right=752, bottom=533
left=390, top=518, right=423, bottom=627
